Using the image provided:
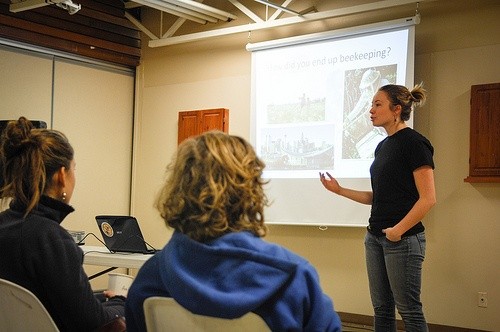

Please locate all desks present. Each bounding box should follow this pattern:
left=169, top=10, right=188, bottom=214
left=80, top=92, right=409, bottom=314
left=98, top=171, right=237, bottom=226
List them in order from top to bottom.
left=79, top=244, right=157, bottom=269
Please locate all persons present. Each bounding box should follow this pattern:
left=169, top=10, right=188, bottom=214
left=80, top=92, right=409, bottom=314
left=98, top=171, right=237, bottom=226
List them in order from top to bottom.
left=348, top=69, right=391, bottom=122
left=319, top=84, right=435, bottom=332
left=0, top=116, right=127, bottom=331
left=126, top=129, right=343, bottom=332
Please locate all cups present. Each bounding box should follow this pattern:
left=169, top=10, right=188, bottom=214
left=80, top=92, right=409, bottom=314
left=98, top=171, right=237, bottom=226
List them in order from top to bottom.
left=105, top=272, right=134, bottom=303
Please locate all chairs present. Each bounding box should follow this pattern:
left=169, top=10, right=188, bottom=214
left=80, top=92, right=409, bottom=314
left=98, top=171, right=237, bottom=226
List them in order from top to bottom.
left=0, top=278, right=60, bottom=332
left=143, top=296, right=271, bottom=332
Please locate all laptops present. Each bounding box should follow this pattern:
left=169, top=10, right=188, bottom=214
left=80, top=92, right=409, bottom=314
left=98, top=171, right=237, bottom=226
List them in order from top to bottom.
left=95, top=214, right=161, bottom=254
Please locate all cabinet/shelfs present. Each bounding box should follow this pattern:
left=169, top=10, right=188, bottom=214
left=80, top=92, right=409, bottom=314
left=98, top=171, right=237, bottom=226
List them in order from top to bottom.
left=464, top=82, right=500, bottom=182
left=178, top=108, right=229, bottom=146
